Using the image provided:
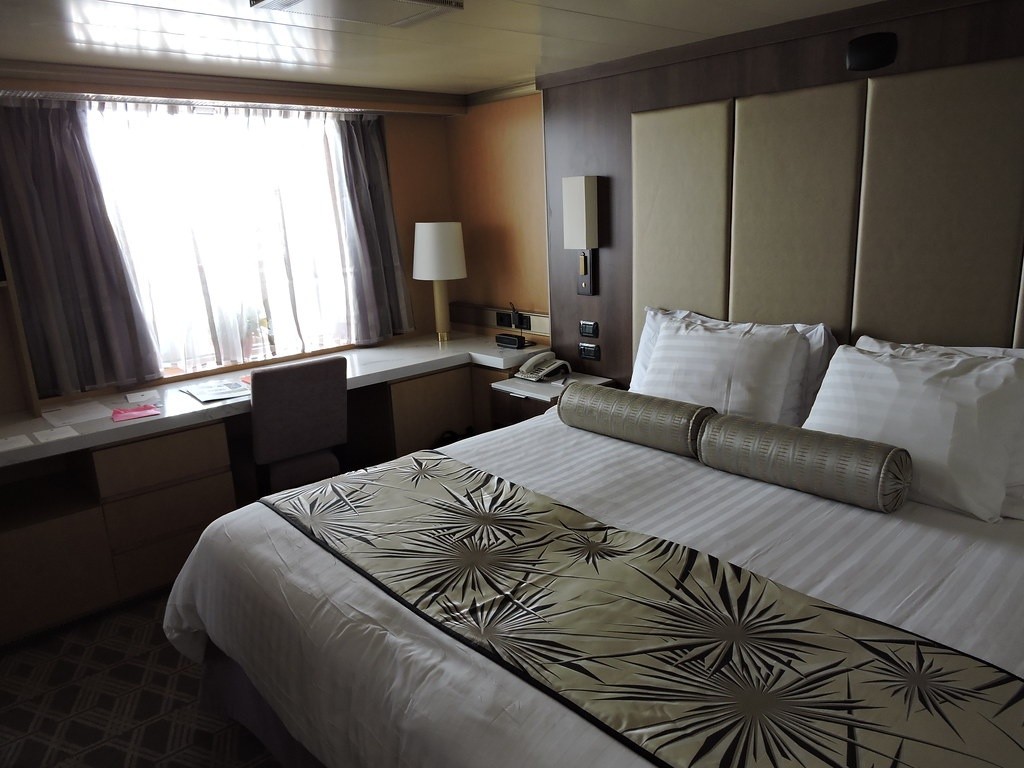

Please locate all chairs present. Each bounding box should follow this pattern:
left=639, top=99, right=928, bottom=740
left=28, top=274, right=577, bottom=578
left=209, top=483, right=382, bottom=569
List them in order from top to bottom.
left=248, top=355, right=351, bottom=494
left=159, top=406, right=1024, bottom=768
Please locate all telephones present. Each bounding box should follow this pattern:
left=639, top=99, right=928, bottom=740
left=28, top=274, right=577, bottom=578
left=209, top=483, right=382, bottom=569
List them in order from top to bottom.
left=515, top=351, right=572, bottom=383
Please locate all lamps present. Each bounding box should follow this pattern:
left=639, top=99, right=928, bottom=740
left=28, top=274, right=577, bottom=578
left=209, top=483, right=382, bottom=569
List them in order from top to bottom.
left=559, top=175, right=600, bottom=297
left=412, top=221, right=470, bottom=341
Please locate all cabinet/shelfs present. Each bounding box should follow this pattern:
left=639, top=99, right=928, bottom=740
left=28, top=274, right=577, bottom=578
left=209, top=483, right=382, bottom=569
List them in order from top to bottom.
left=347, top=360, right=474, bottom=469
left=469, top=362, right=521, bottom=436
left=87, top=418, right=237, bottom=600
left=1, top=462, right=120, bottom=645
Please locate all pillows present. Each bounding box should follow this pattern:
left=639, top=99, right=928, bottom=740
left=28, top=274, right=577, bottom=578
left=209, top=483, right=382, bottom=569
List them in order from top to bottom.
left=628, top=305, right=837, bottom=419
left=641, top=315, right=811, bottom=427
left=856, top=334, right=1023, bottom=520
left=801, top=343, right=1024, bottom=526
left=555, top=381, right=719, bottom=457
left=697, top=412, right=913, bottom=515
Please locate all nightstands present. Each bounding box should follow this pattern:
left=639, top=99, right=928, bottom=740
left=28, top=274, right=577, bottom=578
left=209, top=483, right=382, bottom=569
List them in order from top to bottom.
left=490, top=370, right=614, bottom=429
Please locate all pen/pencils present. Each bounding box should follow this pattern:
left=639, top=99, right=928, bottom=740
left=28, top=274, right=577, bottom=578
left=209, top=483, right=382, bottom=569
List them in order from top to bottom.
left=562, top=377, right=567, bottom=384
